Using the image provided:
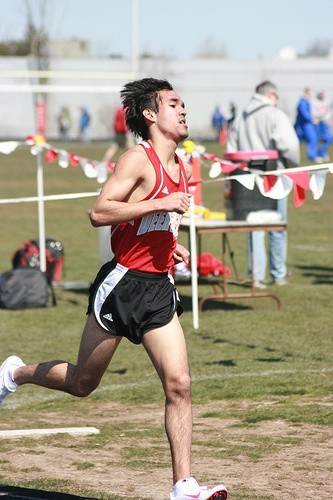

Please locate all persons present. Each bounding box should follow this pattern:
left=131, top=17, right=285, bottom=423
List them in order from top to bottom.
left=0, top=78, right=230, bottom=500
left=222, top=80, right=301, bottom=288
left=295, top=88, right=325, bottom=164
left=312, top=90, right=332, bottom=162
left=102, top=104, right=136, bottom=163
left=212, top=105, right=226, bottom=141
left=54, top=106, right=71, bottom=141
left=77, top=106, right=90, bottom=142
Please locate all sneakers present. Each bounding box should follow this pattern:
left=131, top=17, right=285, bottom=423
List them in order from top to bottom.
left=0, top=355, right=25, bottom=405
left=169, top=477, right=227, bottom=500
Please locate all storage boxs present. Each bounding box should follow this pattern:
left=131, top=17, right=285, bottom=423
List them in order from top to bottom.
left=223, top=151, right=280, bottom=220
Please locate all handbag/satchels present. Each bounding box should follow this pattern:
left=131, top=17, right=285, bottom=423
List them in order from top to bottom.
left=11, top=238, right=63, bottom=283
left=0, top=267, right=55, bottom=309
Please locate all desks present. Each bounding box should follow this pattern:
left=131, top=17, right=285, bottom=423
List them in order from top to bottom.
left=179, top=218, right=288, bottom=316
